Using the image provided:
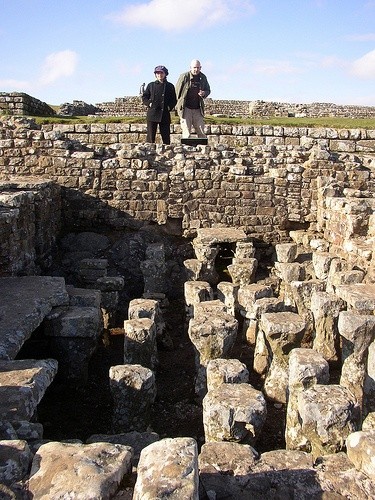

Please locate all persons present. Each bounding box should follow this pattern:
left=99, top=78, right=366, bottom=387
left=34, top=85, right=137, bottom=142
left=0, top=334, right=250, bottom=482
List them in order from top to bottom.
left=174, top=59, right=210, bottom=139
left=142, top=66, right=177, bottom=145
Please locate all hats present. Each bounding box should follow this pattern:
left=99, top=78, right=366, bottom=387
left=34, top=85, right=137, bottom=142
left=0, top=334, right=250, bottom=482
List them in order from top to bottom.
left=154, top=65, right=168, bottom=75
